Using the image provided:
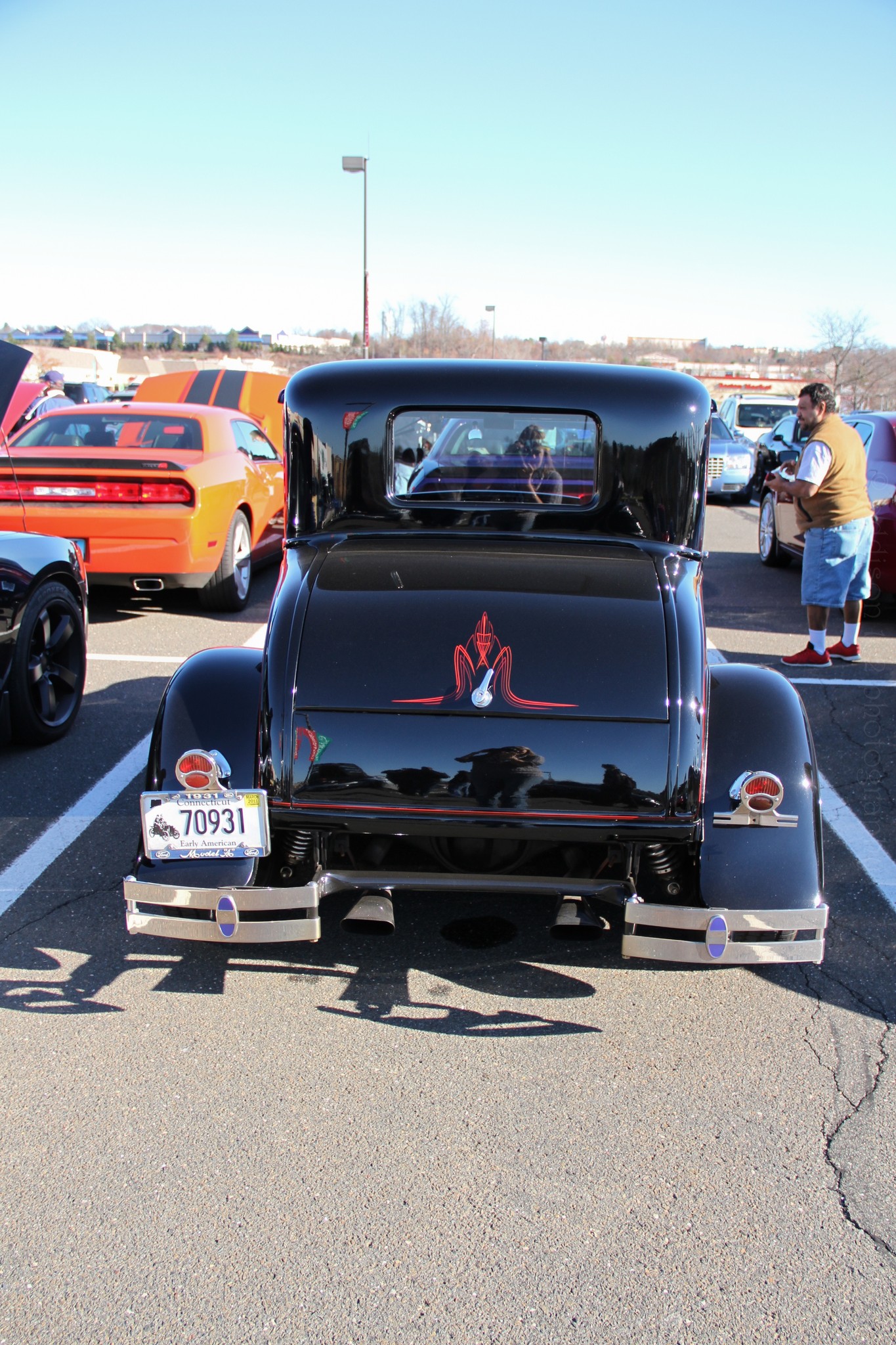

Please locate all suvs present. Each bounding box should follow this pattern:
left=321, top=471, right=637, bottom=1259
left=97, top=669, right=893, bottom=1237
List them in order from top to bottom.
left=721, top=391, right=808, bottom=458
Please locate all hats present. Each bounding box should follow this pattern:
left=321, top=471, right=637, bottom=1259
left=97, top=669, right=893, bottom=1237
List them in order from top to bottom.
left=39, top=371, right=64, bottom=384
left=516, top=423, right=551, bottom=451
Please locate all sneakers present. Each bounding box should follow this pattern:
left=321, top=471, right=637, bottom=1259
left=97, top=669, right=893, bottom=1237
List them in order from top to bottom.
left=826, top=635, right=861, bottom=662
left=780, top=641, right=833, bottom=667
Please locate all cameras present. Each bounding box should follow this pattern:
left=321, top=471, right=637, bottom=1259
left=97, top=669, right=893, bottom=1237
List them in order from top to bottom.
left=515, top=442, right=531, bottom=460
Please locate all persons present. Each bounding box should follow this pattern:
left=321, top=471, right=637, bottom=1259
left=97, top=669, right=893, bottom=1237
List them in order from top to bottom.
left=18, top=370, right=81, bottom=440
left=763, top=382, right=876, bottom=671
left=264, top=396, right=723, bottom=830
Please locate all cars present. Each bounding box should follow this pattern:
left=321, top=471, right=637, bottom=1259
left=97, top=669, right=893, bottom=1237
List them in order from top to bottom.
left=120, top=352, right=832, bottom=978
left=1, top=361, right=285, bottom=601
left=756, top=404, right=895, bottom=607
left=756, top=409, right=811, bottom=480
left=706, top=416, right=754, bottom=498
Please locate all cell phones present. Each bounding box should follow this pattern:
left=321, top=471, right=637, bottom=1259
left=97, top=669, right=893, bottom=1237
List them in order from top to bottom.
left=765, top=473, right=789, bottom=484
